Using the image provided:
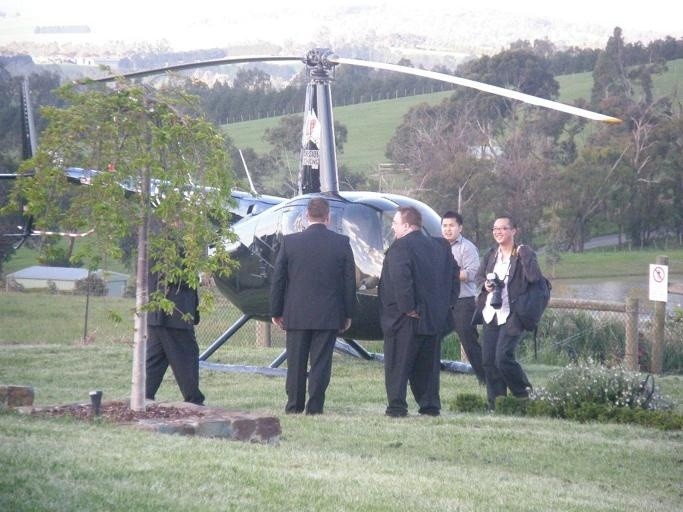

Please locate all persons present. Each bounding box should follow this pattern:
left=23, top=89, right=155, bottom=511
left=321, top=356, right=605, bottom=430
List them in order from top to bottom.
left=269, top=196, right=355, bottom=415
left=144, top=206, right=214, bottom=407
left=378, top=206, right=461, bottom=418
left=439, top=210, right=488, bottom=388
left=470, top=216, right=541, bottom=412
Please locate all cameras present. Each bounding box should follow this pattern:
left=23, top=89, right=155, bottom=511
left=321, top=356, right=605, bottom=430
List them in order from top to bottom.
left=487, top=273, right=505, bottom=309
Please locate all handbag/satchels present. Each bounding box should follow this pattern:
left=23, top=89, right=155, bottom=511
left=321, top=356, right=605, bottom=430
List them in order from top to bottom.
left=512, top=276, right=552, bottom=331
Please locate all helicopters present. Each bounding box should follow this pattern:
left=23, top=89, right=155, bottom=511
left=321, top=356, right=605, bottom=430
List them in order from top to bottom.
left=0, top=48, right=623, bottom=377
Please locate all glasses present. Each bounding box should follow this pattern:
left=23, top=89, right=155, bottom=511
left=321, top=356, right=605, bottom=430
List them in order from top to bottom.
left=492, top=227, right=508, bottom=231
